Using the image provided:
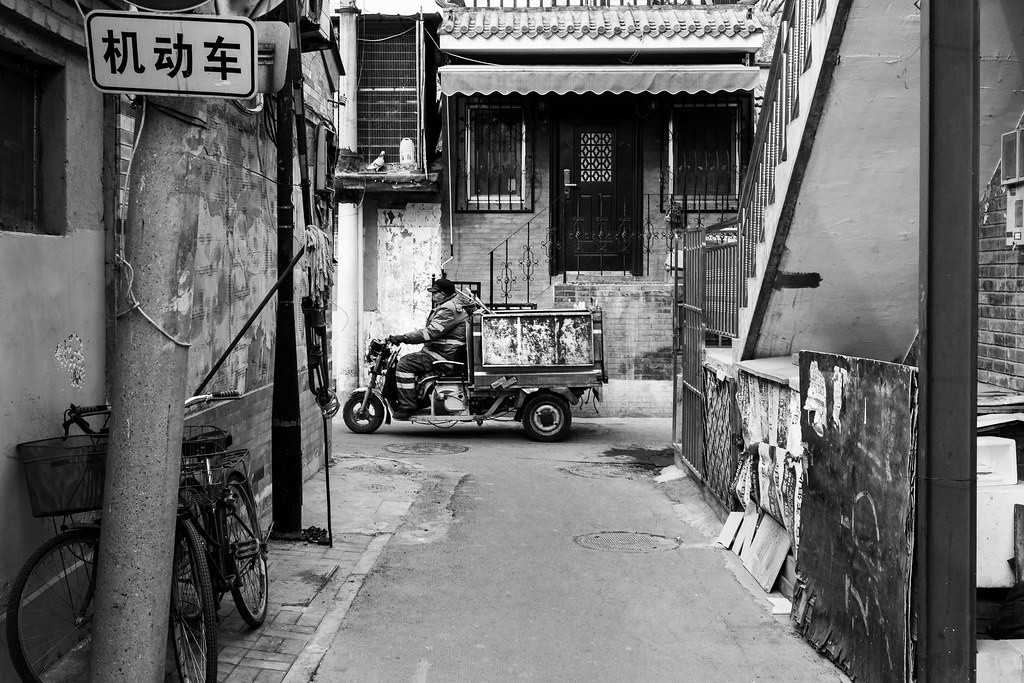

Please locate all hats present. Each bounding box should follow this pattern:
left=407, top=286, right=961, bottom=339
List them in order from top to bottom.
left=427, top=278, right=455, bottom=298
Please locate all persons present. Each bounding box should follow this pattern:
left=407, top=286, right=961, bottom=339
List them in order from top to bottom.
left=383, top=278, right=466, bottom=415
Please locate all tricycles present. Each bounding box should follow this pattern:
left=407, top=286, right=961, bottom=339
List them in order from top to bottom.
left=346, top=300, right=610, bottom=442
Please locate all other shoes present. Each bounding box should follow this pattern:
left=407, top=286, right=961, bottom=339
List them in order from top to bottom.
left=415, top=393, right=431, bottom=410
left=392, top=407, right=416, bottom=419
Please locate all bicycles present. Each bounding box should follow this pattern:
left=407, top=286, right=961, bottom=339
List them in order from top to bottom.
left=6, top=388, right=269, bottom=682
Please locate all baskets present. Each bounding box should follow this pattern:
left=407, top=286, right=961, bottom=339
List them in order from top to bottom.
left=176, top=425, right=228, bottom=504
left=16, top=433, right=108, bottom=518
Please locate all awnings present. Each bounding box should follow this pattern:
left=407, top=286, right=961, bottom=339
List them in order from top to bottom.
left=437, top=63, right=761, bottom=96
